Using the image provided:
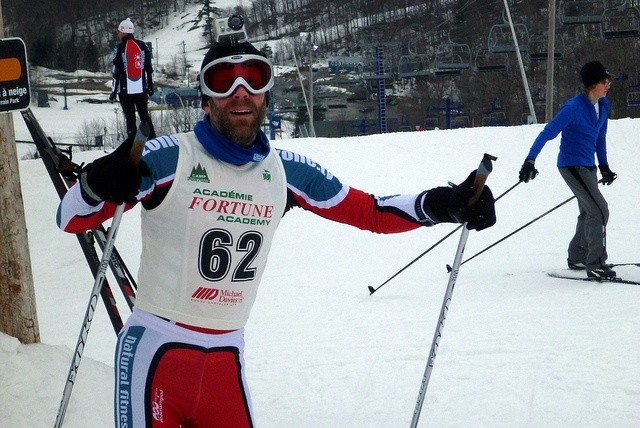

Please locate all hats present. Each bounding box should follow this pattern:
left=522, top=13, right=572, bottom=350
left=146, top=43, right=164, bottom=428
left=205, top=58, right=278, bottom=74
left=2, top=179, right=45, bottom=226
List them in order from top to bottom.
left=117, top=17, right=134, bottom=33
left=199, top=41, right=270, bottom=109
left=580, top=61, right=611, bottom=86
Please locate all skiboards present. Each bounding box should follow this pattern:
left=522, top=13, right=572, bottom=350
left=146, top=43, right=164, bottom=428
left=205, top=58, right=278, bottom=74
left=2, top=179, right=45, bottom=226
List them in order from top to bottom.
left=47, top=136, right=137, bottom=337
left=547, top=263, right=640, bottom=285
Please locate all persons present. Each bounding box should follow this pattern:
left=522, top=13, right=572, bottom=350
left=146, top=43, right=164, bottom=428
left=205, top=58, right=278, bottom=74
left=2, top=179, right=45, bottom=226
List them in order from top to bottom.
left=109, top=17, right=157, bottom=140
left=55, top=40, right=497, bottom=428
left=518, top=59, right=617, bottom=280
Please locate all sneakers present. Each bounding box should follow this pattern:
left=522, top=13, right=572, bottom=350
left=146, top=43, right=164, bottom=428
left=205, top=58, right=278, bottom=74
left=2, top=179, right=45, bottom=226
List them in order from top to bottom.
left=568, top=263, right=587, bottom=269
left=587, top=268, right=616, bottom=278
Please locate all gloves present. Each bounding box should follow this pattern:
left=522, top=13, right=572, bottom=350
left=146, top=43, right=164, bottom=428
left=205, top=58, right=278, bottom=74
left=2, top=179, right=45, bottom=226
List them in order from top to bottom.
left=147, top=85, right=154, bottom=97
left=109, top=92, right=118, bottom=103
left=76, top=136, right=142, bottom=206
left=519, top=158, right=536, bottom=183
left=598, top=164, right=613, bottom=185
left=423, top=170, right=495, bottom=231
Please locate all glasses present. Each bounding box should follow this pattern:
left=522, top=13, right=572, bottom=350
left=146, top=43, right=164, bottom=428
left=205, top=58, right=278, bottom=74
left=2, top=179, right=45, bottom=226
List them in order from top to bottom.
left=196, top=54, right=274, bottom=98
left=600, top=79, right=612, bottom=86
